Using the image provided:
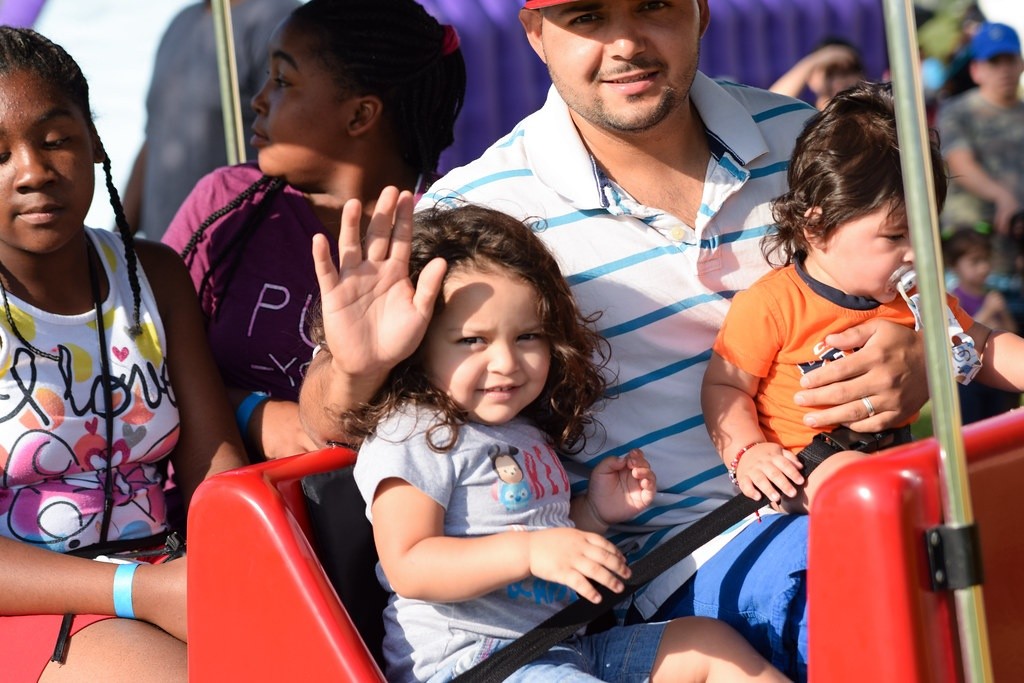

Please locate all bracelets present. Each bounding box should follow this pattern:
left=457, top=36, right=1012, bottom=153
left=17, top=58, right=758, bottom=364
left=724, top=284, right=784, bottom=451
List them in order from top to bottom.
left=236, top=391, right=270, bottom=432
left=728, top=440, right=765, bottom=487
left=113, top=563, right=141, bottom=619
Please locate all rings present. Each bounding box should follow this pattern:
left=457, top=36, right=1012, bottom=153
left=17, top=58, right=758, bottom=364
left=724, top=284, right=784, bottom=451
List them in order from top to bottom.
left=862, top=397, right=875, bottom=417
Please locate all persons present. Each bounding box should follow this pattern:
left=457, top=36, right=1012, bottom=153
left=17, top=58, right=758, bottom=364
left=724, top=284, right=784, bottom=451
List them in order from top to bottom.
left=0, top=0, right=1024, bottom=683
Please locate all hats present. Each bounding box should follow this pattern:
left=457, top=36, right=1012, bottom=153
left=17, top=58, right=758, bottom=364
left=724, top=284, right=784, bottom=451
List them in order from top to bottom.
left=524, top=0, right=581, bottom=10
left=971, top=23, right=1020, bottom=61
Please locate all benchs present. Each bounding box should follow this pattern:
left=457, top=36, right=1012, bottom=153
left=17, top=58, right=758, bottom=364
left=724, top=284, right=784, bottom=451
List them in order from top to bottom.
left=805, top=408, right=1024, bottom=683
left=186, top=443, right=399, bottom=683
